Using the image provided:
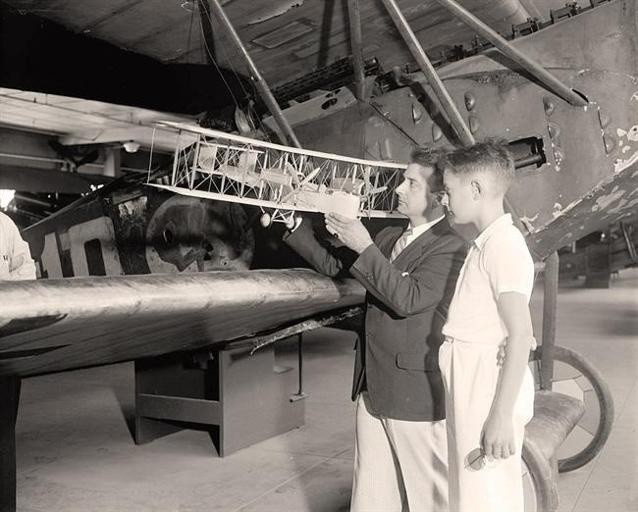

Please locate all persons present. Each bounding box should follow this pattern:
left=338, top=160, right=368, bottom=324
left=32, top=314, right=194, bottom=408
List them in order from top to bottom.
left=433, top=138, right=537, bottom=512
left=283, top=142, right=470, bottom=512
left=0, top=211, right=37, bottom=512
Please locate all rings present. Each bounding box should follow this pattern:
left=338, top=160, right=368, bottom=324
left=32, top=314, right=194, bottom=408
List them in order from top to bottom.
left=334, top=232, right=339, bottom=238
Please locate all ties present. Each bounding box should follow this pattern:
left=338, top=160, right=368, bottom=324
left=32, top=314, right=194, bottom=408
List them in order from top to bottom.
left=390, top=230, right=413, bottom=260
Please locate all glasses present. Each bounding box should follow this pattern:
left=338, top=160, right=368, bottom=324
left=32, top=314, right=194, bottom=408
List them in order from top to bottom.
left=463, top=450, right=489, bottom=472
